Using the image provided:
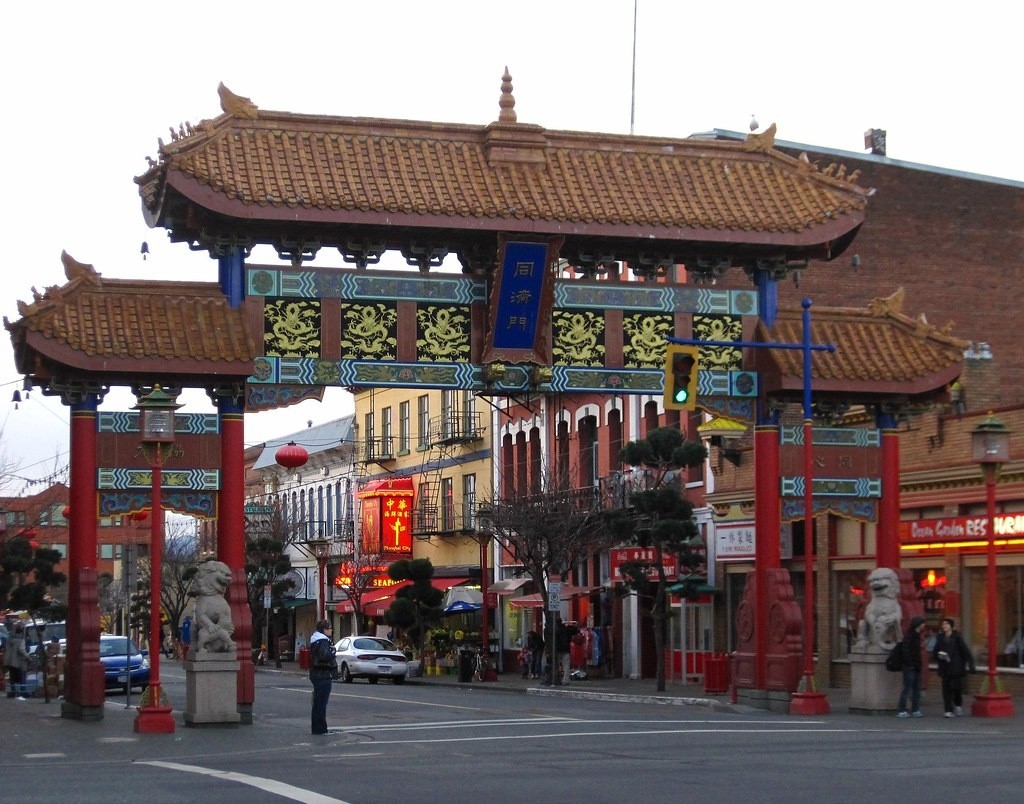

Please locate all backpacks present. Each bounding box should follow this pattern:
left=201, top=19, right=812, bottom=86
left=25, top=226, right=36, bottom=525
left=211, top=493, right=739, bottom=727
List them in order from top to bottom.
left=884, top=642, right=905, bottom=672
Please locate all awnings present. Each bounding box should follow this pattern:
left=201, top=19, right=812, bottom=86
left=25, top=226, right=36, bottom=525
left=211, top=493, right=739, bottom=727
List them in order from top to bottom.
left=488, top=578, right=537, bottom=594
left=336, top=579, right=470, bottom=617
left=510, top=584, right=604, bottom=610
left=279, top=601, right=313, bottom=614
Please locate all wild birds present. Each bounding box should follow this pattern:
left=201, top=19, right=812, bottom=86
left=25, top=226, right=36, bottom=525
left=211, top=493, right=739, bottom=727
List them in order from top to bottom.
left=749, top=113, right=759, bottom=130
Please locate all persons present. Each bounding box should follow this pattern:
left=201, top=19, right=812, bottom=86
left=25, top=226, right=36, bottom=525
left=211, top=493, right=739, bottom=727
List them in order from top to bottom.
left=886, top=617, right=927, bottom=719
left=932, top=618, right=976, bottom=718
left=308, top=619, right=340, bottom=735
left=3, top=623, right=32, bottom=695
left=527, top=629, right=545, bottom=679
left=517, top=647, right=531, bottom=680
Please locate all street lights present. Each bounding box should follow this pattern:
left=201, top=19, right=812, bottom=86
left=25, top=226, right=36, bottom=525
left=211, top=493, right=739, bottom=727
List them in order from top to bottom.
left=664, top=344, right=698, bottom=412
left=128, top=382, right=188, bottom=736
left=964, top=410, right=1017, bottom=718
left=472, top=503, right=498, bottom=683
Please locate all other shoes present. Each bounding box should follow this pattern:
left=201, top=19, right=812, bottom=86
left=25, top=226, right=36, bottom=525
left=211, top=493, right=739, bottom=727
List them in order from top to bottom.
left=955, top=707, right=963, bottom=716
left=897, top=711, right=910, bottom=719
left=910, top=711, right=925, bottom=718
left=943, top=711, right=954, bottom=719
left=312, top=729, right=335, bottom=736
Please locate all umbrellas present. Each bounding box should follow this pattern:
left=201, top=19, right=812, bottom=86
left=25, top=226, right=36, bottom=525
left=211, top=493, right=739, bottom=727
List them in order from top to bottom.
left=437, top=587, right=485, bottom=635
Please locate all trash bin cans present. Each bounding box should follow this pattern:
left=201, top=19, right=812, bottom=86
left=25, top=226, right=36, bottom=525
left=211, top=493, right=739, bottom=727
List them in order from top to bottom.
left=300, top=647, right=310, bottom=669
left=458, top=650, right=475, bottom=682
left=702, top=652, right=728, bottom=692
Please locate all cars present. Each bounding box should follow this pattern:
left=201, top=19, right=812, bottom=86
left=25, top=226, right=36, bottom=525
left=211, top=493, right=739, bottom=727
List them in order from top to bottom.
left=20, top=617, right=67, bottom=695
left=333, top=635, right=410, bottom=685
left=100, top=636, right=151, bottom=694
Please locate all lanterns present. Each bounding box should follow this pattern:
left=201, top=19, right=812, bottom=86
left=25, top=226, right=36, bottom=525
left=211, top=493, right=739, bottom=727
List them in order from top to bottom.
left=275, top=444, right=309, bottom=473
left=61, top=506, right=71, bottom=518
left=129, top=510, right=148, bottom=520
left=17, top=526, right=36, bottom=538
left=28, top=540, right=40, bottom=547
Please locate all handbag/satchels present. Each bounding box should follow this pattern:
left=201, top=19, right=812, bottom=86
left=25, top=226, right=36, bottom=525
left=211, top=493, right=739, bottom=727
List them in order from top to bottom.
left=312, top=654, right=338, bottom=671
